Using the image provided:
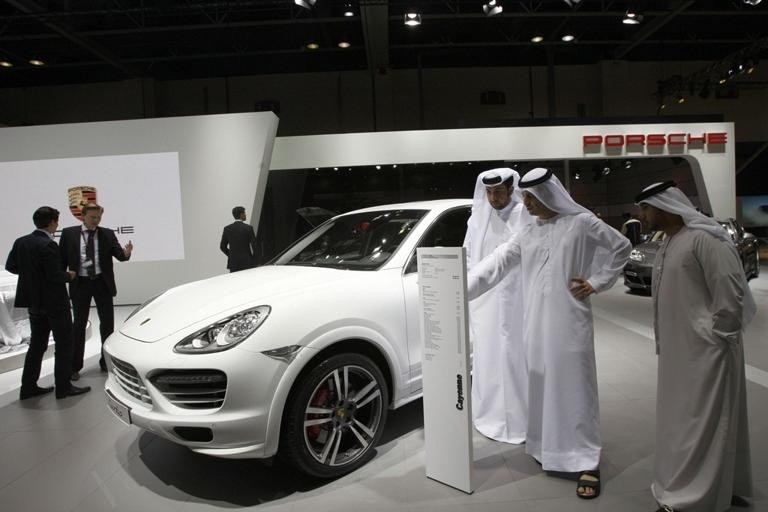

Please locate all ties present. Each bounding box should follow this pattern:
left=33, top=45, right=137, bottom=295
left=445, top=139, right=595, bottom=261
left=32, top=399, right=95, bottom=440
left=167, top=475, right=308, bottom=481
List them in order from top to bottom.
left=86, top=230, right=95, bottom=281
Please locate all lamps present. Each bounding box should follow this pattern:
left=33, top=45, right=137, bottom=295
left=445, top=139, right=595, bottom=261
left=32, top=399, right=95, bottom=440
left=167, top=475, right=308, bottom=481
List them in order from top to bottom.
left=294, top=0, right=318, bottom=10
left=482, top=0, right=502, bottom=19
left=342, top=2, right=354, bottom=18
left=622, top=9, right=644, bottom=27
left=404, top=12, right=422, bottom=27
left=625, top=159, right=632, bottom=169
left=602, top=166, right=613, bottom=177
left=575, top=171, right=582, bottom=181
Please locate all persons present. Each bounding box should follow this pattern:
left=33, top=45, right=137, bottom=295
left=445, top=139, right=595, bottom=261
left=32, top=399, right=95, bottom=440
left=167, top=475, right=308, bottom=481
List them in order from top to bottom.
left=464, top=167, right=533, bottom=445
left=464, top=169, right=633, bottom=496
left=634, top=177, right=753, bottom=511
left=60, top=203, right=134, bottom=378
left=4, top=205, right=92, bottom=399
left=219, top=206, right=261, bottom=273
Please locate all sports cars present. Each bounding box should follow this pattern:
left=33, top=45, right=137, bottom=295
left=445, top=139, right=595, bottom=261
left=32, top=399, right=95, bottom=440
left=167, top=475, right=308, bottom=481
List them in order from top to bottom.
left=620, top=215, right=762, bottom=295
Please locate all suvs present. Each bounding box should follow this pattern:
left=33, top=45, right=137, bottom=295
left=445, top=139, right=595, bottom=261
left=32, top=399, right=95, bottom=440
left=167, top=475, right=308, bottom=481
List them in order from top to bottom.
left=96, top=197, right=477, bottom=478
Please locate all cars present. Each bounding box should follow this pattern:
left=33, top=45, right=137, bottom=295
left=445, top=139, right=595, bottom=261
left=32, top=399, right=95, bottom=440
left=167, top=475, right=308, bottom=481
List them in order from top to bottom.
left=295, top=205, right=364, bottom=261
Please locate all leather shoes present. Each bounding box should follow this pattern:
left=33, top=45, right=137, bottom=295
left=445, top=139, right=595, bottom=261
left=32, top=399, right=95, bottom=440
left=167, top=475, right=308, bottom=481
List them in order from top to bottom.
left=54, top=371, right=90, bottom=399
left=20, top=384, right=54, bottom=400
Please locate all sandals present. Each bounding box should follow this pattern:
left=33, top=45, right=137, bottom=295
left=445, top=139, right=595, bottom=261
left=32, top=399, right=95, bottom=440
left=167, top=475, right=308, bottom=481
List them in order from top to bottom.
left=576, top=470, right=599, bottom=498
